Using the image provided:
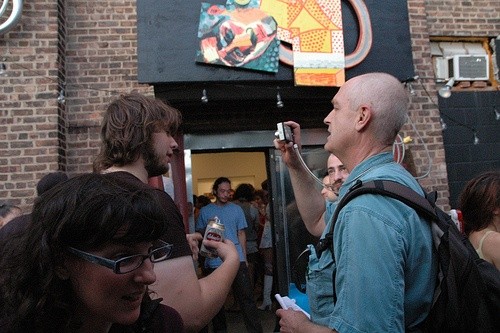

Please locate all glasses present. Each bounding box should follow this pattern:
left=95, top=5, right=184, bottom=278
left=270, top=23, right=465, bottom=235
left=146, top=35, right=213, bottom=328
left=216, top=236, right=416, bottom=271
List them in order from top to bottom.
left=62, top=238, right=174, bottom=273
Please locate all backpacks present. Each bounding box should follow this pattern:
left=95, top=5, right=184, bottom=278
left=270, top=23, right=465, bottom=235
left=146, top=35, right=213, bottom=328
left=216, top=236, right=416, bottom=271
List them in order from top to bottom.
left=326, top=179, right=500, bottom=333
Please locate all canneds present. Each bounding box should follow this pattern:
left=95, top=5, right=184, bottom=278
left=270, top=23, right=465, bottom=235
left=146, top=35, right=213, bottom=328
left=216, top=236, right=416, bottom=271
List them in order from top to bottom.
left=199, top=221, right=225, bottom=258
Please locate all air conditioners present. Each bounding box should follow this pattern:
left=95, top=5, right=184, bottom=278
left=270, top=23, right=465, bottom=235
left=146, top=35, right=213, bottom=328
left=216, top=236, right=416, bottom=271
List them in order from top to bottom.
left=447, top=54, right=491, bottom=82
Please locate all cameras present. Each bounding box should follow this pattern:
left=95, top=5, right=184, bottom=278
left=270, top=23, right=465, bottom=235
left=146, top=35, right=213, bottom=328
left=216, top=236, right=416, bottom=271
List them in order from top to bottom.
left=275, top=122, right=293, bottom=142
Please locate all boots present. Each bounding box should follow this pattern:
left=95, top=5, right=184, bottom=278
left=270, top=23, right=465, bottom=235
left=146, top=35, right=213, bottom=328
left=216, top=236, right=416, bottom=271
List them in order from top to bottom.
left=258, top=274, right=272, bottom=312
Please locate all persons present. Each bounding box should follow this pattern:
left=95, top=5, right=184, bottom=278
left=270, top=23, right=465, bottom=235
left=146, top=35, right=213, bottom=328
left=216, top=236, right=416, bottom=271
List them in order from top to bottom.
left=273, top=72, right=500, bottom=333
left=92, top=92, right=240, bottom=333
left=321, top=152, right=351, bottom=205
left=0, top=171, right=67, bottom=270
left=20, top=171, right=186, bottom=333
left=460, top=171, right=500, bottom=271
left=195, top=176, right=264, bottom=332
left=191, top=180, right=275, bottom=311
left=448, top=207, right=462, bottom=233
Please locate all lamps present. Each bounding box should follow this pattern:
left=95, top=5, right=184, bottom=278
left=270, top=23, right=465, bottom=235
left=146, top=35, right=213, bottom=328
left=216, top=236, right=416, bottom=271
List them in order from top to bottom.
left=440, top=77, right=455, bottom=97
